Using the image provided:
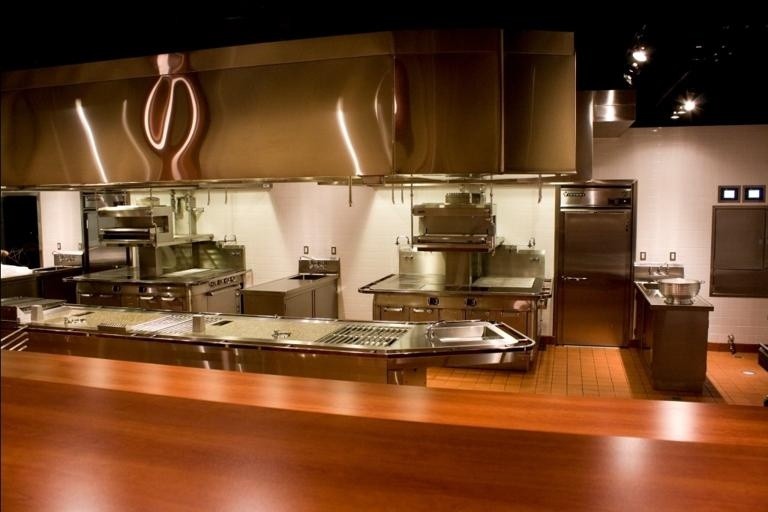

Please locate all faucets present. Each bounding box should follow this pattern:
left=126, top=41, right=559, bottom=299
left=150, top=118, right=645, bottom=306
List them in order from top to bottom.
left=657, top=266, right=662, bottom=272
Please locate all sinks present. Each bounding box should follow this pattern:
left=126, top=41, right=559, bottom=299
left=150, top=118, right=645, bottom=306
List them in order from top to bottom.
left=643, top=281, right=661, bottom=291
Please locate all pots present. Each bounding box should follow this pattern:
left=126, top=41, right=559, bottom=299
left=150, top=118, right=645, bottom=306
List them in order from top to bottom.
left=656, top=279, right=707, bottom=303
